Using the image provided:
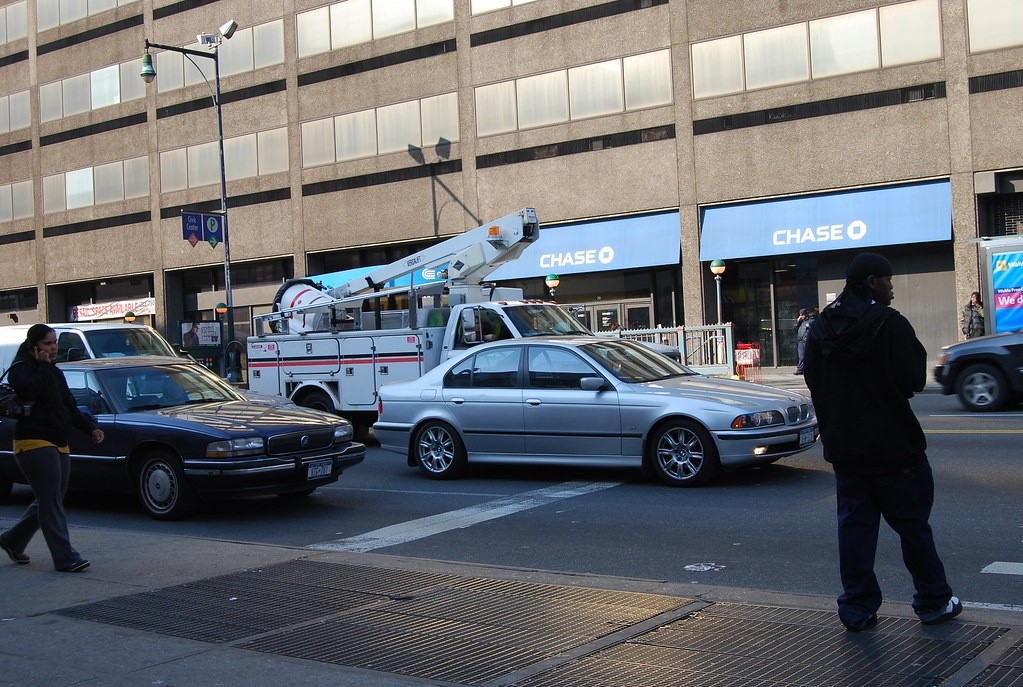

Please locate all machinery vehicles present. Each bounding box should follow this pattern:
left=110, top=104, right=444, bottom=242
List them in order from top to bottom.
left=247, top=206, right=682, bottom=446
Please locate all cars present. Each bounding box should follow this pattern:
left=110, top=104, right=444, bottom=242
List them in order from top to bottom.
left=933, top=324, right=1023, bottom=414
left=372, top=333, right=823, bottom=480
left=0, top=355, right=366, bottom=521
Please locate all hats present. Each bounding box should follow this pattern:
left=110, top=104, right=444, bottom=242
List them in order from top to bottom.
left=845, top=253, right=893, bottom=279
left=799, top=309, right=810, bottom=315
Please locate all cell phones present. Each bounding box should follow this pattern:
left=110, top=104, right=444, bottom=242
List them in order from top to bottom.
left=32, top=344, right=42, bottom=354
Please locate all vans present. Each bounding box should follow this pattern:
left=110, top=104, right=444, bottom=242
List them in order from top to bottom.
left=0, top=321, right=225, bottom=409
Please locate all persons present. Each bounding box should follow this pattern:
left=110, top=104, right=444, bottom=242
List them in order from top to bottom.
left=803, top=254, right=963, bottom=632
left=961, top=291, right=984, bottom=341
left=794, top=305, right=819, bottom=375
left=569, top=308, right=579, bottom=317
left=463, top=317, right=496, bottom=348
left=183, top=322, right=201, bottom=346
left=610, top=322, right=626, bottom=338
left=0, top=324, right=104, bottom=571
left=661, top=337, right=669, bottom=345
left=716, top=337, right=725, bottom=364
left=94, top=375, right=129, bottom=414
left=152, top=378, right=184, bottom=406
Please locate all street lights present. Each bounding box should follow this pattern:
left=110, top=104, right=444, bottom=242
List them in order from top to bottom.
left=139, top=17, right=243, bottom=388
left=709, top=257, right=727, bottom=325
left=545, top=273, right=561, bottom=302
left=215, top=302, right=227, bottom=322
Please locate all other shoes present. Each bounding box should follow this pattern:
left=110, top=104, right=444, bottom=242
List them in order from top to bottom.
left=793, top=372, right=803, bottom=375
left=1, top=534, right=31, bottom=563
left=62, top=559, right=90, bottom=572
left=926, top=596, right=962, bottom=624
left=861, top=613, right=877, bottom=631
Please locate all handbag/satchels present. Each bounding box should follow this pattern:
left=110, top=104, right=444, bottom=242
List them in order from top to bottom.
left=0, top=361, right=34, bottom=420
